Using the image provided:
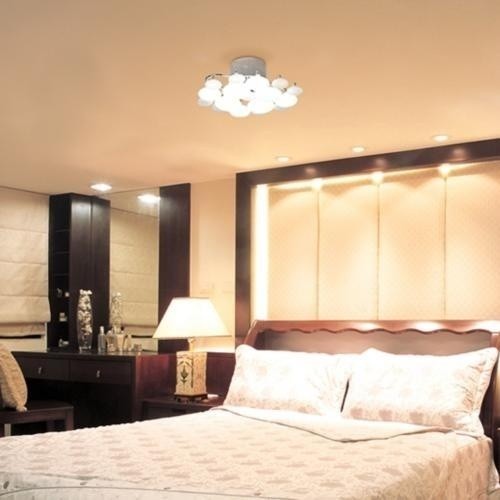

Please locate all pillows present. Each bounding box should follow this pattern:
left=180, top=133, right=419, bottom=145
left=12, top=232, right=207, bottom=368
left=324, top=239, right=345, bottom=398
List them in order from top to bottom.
left=223, top=343, right=500, bottom=435
left=0, top=344, right=29, bottom=412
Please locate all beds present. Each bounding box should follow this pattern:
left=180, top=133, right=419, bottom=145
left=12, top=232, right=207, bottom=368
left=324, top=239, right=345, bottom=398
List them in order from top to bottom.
left=0, top=318, right=500, bottom=499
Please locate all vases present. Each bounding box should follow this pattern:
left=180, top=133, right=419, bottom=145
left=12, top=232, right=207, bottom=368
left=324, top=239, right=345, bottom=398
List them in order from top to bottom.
left=77, top=289, right=93, bottom=351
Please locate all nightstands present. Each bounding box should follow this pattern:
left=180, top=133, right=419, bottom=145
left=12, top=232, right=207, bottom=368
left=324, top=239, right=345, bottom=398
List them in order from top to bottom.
left=140, top=394, right=225, bottom=421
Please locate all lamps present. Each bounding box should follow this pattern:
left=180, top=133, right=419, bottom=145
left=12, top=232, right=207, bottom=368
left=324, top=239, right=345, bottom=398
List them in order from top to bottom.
left=194, top=55, right=304, bottom=119
left=153, top=297, right=231, bottom=403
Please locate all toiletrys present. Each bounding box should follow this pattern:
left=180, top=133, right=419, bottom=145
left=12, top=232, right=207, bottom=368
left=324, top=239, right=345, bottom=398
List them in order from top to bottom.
left=97, top=326, right=133, bottom=353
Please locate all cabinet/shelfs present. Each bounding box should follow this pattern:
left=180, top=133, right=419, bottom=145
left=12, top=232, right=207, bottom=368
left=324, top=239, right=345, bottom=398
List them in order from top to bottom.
left=48, top=193, right=110, bottom=350
left=10, top=349, right=176, bottom=421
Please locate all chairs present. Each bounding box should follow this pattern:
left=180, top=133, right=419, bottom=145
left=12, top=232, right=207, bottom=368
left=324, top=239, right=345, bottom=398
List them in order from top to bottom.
left=0, top=399, right=75, bottom=437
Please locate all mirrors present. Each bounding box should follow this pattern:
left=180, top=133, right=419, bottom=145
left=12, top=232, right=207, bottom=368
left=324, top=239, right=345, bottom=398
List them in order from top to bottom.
left=109, top=189, right=159, bottom=353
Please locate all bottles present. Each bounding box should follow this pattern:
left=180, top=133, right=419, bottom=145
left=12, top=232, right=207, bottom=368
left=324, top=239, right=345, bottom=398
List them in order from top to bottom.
left=97, top=325, right=106, bottom=353
